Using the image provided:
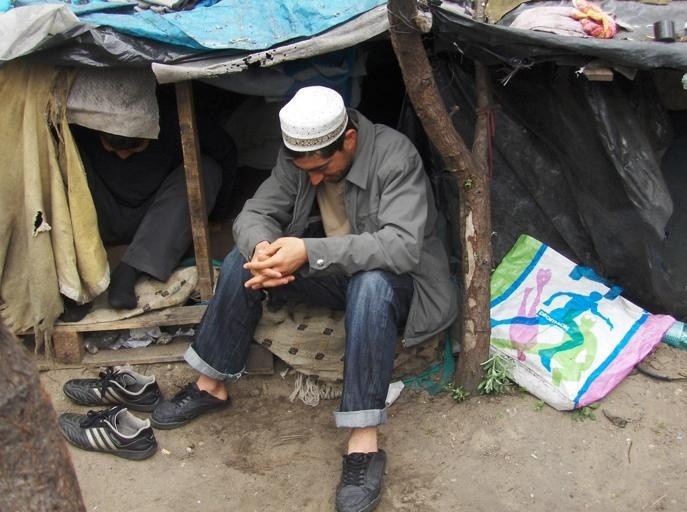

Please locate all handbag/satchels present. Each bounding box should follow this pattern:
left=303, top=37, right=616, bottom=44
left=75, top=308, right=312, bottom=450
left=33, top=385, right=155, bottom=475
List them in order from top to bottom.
left=486, top=233, right=677, bottom=412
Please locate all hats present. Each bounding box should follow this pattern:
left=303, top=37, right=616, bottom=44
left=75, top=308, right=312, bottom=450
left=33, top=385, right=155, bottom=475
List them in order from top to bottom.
left=278, top=85, right=350, bottom=153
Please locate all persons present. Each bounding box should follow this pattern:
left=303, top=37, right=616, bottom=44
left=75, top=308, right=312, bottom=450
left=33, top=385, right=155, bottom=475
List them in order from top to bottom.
left=144, top=84, right=465, bottom=511
left=33, top=89, right=225, bottom=324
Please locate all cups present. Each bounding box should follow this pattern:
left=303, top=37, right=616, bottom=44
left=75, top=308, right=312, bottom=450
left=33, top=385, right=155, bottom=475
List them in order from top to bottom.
left=653, top=20, right=675, bottom=43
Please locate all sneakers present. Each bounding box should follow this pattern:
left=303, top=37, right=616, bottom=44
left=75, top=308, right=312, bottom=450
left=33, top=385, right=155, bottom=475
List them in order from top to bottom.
left=62, top=368, right=161, bottom=413
left=149, top=381, right=232, bottom=429
left=58, top=406, right=158, bottom=462
left=333, top=446, right=387, bottom=511
